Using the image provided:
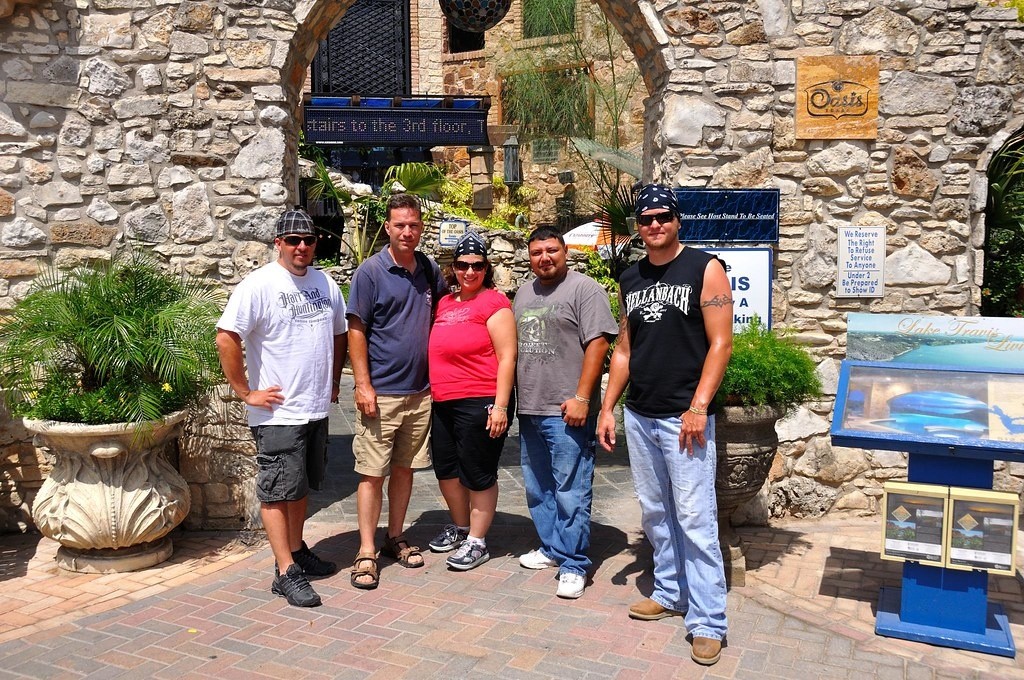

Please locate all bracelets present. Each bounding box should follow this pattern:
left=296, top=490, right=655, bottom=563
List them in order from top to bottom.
left=333, top=379, right=340, bottom=385
left=575, top=395, right=590, bottom=404
left=494, top=405, right=507, bottom=412
left=689, top=406, right=707, bottom=414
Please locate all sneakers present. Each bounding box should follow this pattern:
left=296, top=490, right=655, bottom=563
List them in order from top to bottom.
left=428, top=523, right=469, bottom=552
left=446, top=539, right=490, bottom=570
left=274, top=540, right=338, bottom=579
left=519, top=549, right=559, bottom=569
left=272, top=563, right=321, bottom=607
left=557, top=572, right=587, bottom=598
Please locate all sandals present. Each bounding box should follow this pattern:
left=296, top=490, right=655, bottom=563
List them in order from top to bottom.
left=383, top=530, right=424, bottom=568
left=350, top=552, right=379, bottom=588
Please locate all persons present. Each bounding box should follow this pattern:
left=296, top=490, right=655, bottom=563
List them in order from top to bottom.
left=596, top=184, right=732, bottom=666
left=345, top=194, right=450, bottom=589
left=215, top=210, right=349, bottom=607
left=512, top=226, right=619, bottom=598
left=427, top=232, right=518, bottom=570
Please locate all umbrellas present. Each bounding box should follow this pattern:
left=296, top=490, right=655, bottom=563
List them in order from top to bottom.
left=563, top=219, right=631, bottom=276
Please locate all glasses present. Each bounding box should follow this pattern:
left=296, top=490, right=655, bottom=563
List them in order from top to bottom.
left=636, top=211, right=676, bottom=226
left=279, top=235, right=318, bottom=246
left=452, top=260, right=488, bottom=271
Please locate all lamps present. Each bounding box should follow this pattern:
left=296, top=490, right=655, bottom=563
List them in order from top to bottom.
left=303, top=93, right=491, bottom=110
left=502, top=134, right=520, bottom=185
left=558, top=168, right=576, bottom=185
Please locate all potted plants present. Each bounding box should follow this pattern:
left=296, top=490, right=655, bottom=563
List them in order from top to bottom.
left=0, top=224, right=234, bottom=576
left=716, top=312, right=825, bottom=527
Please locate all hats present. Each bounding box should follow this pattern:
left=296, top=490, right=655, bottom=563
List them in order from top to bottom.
left=276, top=209, right=315, bottom=237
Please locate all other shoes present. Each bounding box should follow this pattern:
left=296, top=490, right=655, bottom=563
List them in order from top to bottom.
left=629, top=597, right=687, bottom=620
left=691, top=636, right=722, bottom=664
